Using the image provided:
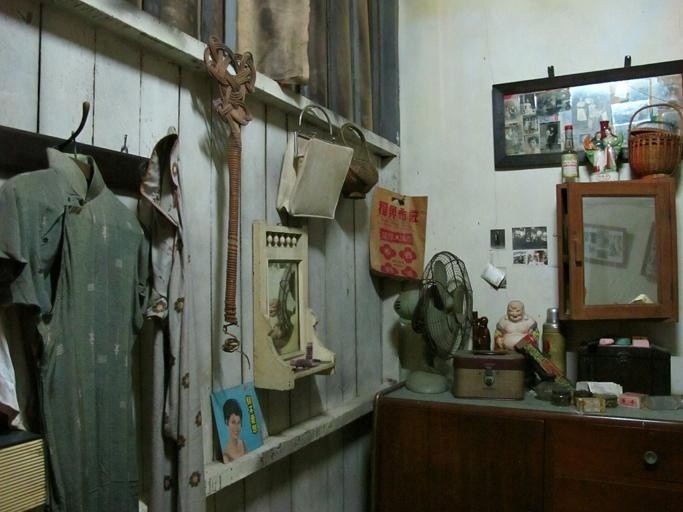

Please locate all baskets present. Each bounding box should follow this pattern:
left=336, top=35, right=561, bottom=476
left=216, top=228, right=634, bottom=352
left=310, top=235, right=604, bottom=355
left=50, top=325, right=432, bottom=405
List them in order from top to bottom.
left=627, top=104, right=681, bottom=179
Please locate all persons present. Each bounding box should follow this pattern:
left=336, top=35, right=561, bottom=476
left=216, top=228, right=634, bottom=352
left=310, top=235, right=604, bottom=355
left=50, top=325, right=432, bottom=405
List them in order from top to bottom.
left=504, top=74, right=683, bottom=155
left=222, top=398, right=247, bottom=464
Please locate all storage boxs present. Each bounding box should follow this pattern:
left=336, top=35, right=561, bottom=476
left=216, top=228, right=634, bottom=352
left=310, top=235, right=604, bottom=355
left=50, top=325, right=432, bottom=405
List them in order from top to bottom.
left=452, top=350, right=526, bottom=401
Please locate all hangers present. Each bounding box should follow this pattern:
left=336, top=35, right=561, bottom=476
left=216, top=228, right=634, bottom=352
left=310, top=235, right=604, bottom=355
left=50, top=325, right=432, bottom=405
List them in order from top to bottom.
left=57, top=129, right=94, bottom=186
left=100, top=134, right=152, bottom=200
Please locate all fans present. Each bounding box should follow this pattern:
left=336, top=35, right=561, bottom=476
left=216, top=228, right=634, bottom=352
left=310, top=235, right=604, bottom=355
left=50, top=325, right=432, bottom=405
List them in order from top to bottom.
left=393, top=250, right=474, bottom=394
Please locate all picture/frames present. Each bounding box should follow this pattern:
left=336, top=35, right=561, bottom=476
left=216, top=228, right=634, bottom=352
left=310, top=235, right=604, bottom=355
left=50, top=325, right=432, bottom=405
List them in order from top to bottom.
left=491, top=59, right=682, bottom=172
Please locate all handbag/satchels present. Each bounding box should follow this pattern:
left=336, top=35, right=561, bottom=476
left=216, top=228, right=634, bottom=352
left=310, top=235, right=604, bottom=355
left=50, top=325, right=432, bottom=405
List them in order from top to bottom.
left=276, top=104, right=354, bottom=221
left=336, top=123, right=379, bottom=200
left=368, top=186, right=428, bottom=282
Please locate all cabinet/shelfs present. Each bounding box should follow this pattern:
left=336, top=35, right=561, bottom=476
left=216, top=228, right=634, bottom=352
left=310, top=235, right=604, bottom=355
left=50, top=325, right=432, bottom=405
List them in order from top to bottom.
left=555, top=175, right=679, bottom=324
left=366, top=382, right=683, bottom=512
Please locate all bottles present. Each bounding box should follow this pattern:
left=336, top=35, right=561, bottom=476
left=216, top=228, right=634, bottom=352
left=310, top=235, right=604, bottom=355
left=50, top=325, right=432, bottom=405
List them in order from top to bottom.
left=559, top=124, right=581, bottom=185
left=541, top=307, right=567, bottom=380
